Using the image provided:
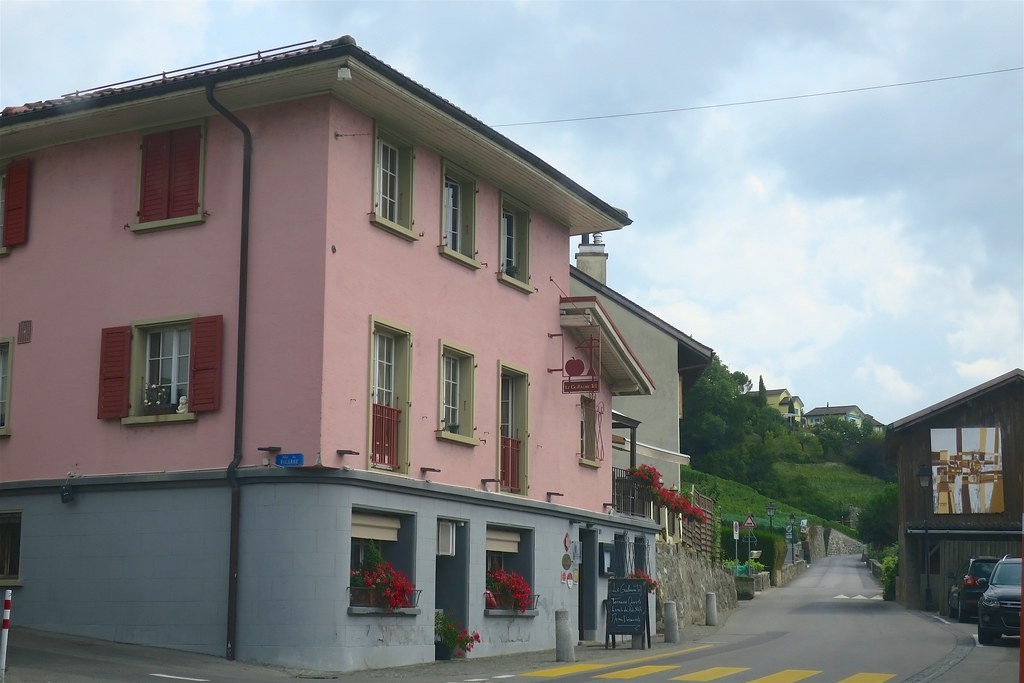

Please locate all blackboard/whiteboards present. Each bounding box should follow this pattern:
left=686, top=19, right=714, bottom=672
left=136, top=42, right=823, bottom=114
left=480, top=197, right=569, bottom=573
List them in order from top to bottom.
left=606, top=578, right=647, bottom=635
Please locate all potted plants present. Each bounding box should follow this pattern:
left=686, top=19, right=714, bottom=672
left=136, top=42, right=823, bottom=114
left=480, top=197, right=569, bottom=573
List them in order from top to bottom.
left=506, top=266, right=520, bottom=277
left=447, top=423, right=459, bottom=434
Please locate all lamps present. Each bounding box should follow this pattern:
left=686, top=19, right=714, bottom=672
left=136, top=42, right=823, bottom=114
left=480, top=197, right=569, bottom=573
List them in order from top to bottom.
left=337, top=67, right=353, bottom=81
left=258, top=446, right=281, bottom=451
left=337, top=450, right=359, bottom=455
left=421, top=467, right=441, bottom=472
left=59, top=473, right=74, bottom=503
left=659, top=478, right=664, bottom=487
left=481, top=479, right=500, bottom=484
left=669, top=484, right=679, bottom=494
left=547, top=492, right=564, bottom=496
left=571, top=541, right=583, bottom=564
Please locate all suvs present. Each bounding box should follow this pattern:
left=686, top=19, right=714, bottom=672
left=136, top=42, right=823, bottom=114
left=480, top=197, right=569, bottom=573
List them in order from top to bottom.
left=975, top=554, right=1022, bottom=645
left=944, top=555, right=1003, bottom=622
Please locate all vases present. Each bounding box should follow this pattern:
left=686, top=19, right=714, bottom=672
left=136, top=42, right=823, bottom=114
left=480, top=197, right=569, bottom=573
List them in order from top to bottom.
left=146, top=404, right=179, bottom=415
left=351, top=587, right=377, bottom=604
left=434, top=640, right=451, bottom=661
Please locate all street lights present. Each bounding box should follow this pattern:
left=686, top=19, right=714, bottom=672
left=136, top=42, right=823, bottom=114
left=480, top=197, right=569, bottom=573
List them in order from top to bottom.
left=916, top=464, right=934, bottom=611
left=764, top=501, right=776, bottom=534
left=790, top=512, right=795, bottom=563
left=841, top=513, right=844, bottom=525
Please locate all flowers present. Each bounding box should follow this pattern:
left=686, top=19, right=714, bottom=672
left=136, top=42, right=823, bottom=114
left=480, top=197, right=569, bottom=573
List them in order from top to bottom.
left=143, top=382, right=172, bottom=408
left=625, top=464, right=707, bottom=524
left=352, top=539, right=416, bottom=612
left=486, top=561, right=533, bottom=614
left=630, top=568, right=660, bottom=595
left=435, top=607, right=481, bottom=658
